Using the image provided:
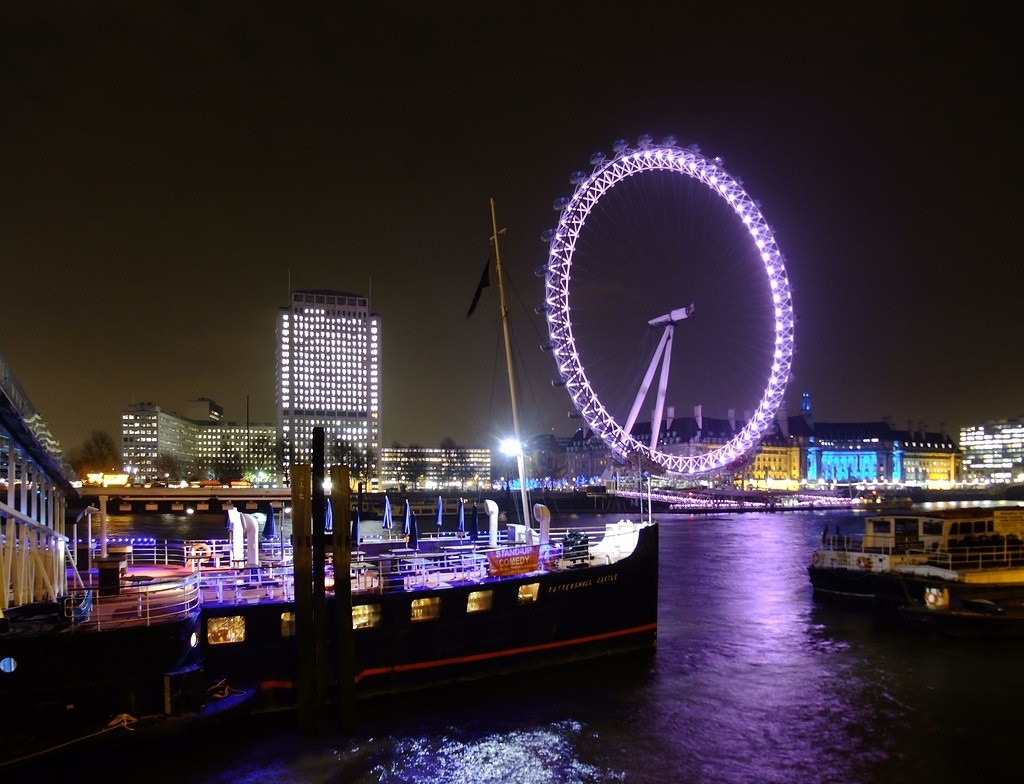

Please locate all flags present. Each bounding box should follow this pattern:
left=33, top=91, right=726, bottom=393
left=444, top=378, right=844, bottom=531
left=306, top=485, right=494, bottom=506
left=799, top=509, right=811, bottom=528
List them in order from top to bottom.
left=466, top=258, right=491, bottom=318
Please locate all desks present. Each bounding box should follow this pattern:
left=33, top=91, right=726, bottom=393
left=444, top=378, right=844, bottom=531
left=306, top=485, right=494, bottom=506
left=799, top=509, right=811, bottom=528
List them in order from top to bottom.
left=351, top=544, right=486, bottom=594
left=184, top=553, right=212, bottom=577
left=230, top=558, right=248, bottom=568
left=122, top=573, right=154, bottom=616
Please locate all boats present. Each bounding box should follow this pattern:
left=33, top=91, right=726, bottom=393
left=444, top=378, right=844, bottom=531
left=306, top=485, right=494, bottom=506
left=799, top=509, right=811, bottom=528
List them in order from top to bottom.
left=2, top=492, right=661, bottom=742
left=807, top=502, right=1023, bottom=630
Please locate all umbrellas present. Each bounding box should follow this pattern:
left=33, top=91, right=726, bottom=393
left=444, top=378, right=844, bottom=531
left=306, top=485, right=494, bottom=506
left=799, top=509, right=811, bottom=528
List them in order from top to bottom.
left=226, top=496, right=478, bottom=586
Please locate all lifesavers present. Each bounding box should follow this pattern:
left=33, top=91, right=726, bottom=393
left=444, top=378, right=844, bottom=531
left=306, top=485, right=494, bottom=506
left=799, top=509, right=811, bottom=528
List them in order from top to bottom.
left=191, top=544, right=211, bottom=563
left=812, top=552, right=819, bottom=564
left=856, top=556, right=867, bottom=567
left=926, top=592, right=937, bottom=604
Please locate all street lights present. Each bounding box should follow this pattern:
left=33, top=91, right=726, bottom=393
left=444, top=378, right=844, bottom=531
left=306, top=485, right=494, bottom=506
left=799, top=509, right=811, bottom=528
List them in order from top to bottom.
left=502, top=437, right=523, bottom=520
